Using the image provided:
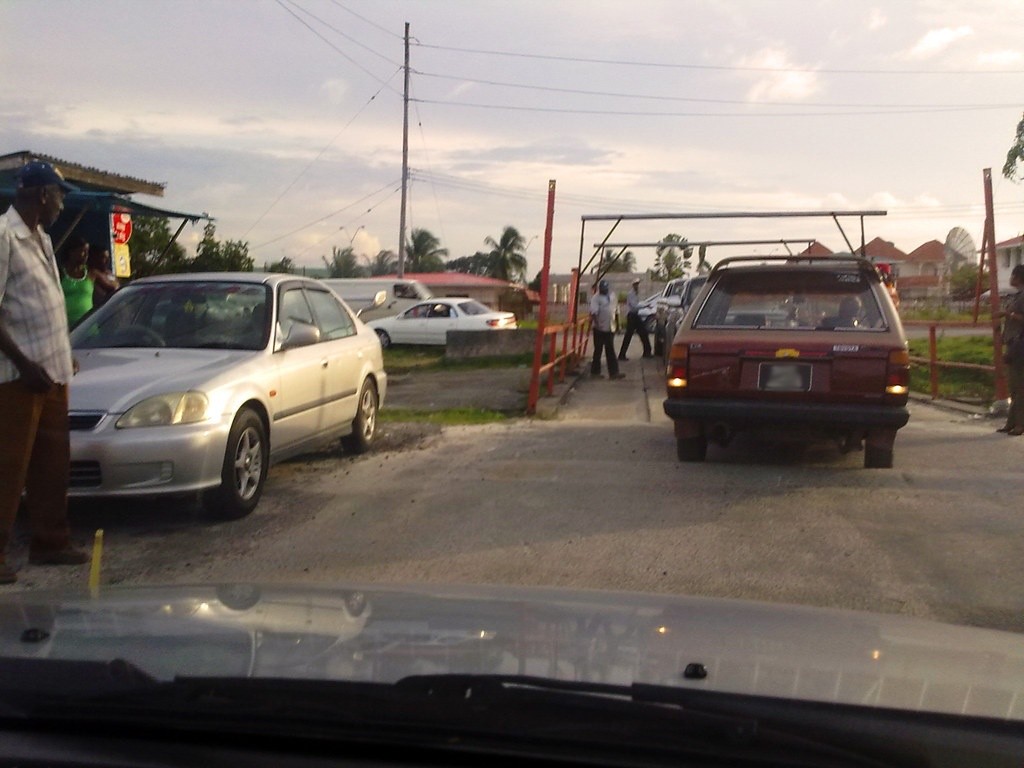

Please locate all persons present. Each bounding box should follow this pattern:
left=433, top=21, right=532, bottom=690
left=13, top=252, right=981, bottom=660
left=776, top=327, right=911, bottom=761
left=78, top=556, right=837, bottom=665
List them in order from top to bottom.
left=0, top=160, right=92, bottom=585
left=60, top=240, right=125, bottom=340
left=991, top=265, right=1024, bottom=435
left=620, top=278, right=655, bottom=361
left=590, top=281, right=626, bottom=379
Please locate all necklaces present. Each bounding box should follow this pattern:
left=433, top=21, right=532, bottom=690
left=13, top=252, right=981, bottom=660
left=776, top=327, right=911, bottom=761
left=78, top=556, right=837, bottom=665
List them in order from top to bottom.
left=63, top=267, right=88, bottom=284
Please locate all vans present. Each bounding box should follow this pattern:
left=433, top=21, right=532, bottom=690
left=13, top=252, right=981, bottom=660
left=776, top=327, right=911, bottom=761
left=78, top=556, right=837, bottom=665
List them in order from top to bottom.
left=320, top=279, right=434, bottom=325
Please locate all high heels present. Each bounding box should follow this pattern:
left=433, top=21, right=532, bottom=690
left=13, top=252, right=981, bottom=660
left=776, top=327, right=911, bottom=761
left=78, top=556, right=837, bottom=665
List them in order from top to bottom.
left=996, top=424, right=1024, bottom=436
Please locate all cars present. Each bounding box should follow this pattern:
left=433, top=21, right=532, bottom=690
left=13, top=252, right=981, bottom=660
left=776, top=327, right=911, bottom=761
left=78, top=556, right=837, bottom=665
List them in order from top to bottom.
left=637, top=256, right=911, bottom=467
left=21, top=271, right=388, bottom=522
left=365, top=297, right=517, bottom=350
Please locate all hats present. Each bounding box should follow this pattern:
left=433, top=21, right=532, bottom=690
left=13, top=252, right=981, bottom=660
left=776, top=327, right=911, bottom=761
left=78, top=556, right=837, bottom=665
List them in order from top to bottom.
left=14, top=160, right=82, bottom=192
left=632, top=277, right=644, bottom=285
left=599, top=280, right=609, bottom=288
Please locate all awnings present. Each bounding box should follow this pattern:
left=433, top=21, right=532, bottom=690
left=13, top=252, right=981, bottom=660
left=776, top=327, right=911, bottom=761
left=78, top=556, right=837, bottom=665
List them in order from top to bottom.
left=1, top=182, right=208, bottom=276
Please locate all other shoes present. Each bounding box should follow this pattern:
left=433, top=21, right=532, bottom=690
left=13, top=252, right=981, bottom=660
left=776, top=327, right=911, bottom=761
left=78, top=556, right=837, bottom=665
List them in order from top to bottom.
left=641, top=353, right=656, bottom=359
left=618, top=355, right=630, bottom=362
left=590, top=371, right=604, bottom=379
left=609, top=372, right=626, bottom=380
left=28, top=542, right=90, bottom=565
left=1, top=560, right=18, bottom=585
